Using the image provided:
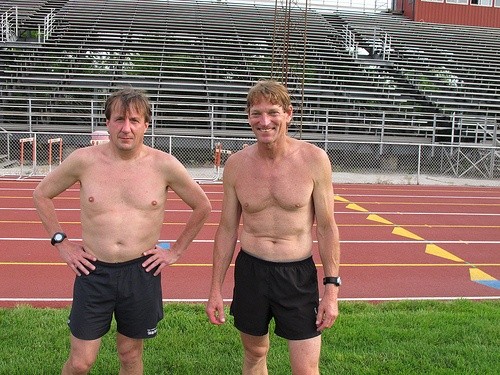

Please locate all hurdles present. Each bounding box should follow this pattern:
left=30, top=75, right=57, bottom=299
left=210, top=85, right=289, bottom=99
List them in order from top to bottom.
left=18, top=137, right=36, bottom=181
left=48, top=138, right=62, bottom=174
left=212, top=143, right=221, bottom=182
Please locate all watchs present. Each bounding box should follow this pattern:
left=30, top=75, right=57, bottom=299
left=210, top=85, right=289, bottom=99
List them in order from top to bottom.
left=50, top=232, right=67, bottom=246
left=322, top=275, right=341, bottom=287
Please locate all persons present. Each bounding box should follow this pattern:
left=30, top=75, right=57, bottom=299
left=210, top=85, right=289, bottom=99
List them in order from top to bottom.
left=205, top=79, right=343, bottom=374
left=32, top=88, right=213, bottom=373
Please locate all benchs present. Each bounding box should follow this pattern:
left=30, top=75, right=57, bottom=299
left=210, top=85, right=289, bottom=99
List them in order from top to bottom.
left=0, top=0, right=500, bottom=181
